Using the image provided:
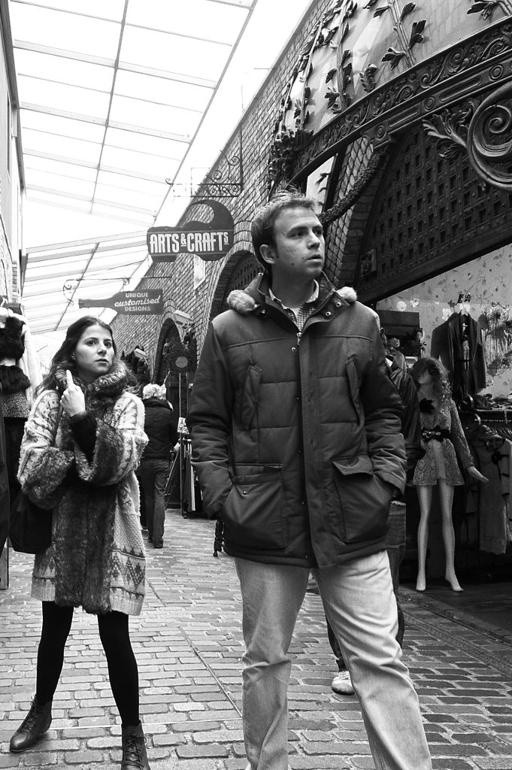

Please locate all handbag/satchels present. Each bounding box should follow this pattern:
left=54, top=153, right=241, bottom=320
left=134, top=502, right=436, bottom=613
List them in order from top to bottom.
left=9, top=479, right=56, bottom=553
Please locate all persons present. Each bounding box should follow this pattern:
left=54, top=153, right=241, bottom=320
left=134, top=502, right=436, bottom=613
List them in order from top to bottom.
left=184, top=195, right=434, bottom=769
left=8, top=314, right=154, bottom=769
left=326, top=354, right=414, bottom=694
left=404, top=356, right=489, bottom=593
left=134, top=382, right=181, bottom=549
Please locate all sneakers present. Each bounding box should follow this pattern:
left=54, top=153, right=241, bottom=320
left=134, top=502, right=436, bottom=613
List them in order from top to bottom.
left=141, top=527, right=151, bottom=540
left=148, top=535, right=163, bottom=548
left=331, top=666, right=359, bottom=696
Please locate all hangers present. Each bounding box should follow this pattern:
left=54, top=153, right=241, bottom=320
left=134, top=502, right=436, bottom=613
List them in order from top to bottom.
left=453, top=291, right=471, bottom=327
left=490, top=300, right=508, bottom=310
left=462, top=412, right=512, bottom=441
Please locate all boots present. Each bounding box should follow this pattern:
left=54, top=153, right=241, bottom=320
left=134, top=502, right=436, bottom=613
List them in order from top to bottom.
left=8, top=693, right=53, bottom=754
left=120, top=720, right=151, bottom=770
